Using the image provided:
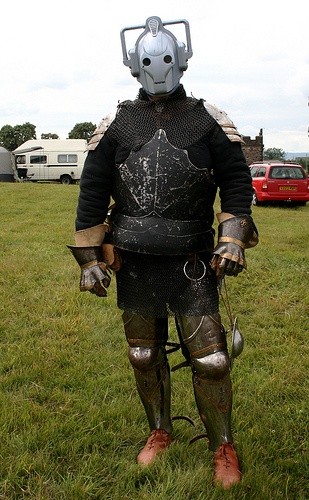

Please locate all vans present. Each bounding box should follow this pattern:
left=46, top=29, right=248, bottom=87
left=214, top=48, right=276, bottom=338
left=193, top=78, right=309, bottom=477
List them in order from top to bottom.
left=10, top=138, right=89, bottom=185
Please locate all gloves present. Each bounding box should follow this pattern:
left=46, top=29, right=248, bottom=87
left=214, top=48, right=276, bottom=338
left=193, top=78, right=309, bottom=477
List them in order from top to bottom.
left=210, top=211, right=259, bottom=279
left=64, top=244, right=112, bottom=298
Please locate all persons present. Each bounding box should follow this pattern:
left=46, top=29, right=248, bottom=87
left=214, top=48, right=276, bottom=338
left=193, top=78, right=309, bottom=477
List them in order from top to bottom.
left=68, top=16, right=260, bottom=490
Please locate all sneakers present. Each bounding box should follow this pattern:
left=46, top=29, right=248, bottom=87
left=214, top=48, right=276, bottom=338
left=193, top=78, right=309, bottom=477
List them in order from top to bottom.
left=137, top=428, right=170, bottom=465
left=212, top=442, right=240, bottom=490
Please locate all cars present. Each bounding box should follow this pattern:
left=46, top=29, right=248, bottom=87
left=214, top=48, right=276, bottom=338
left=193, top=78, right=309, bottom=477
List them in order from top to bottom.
left=248, top=160, right=309, bottom=206
